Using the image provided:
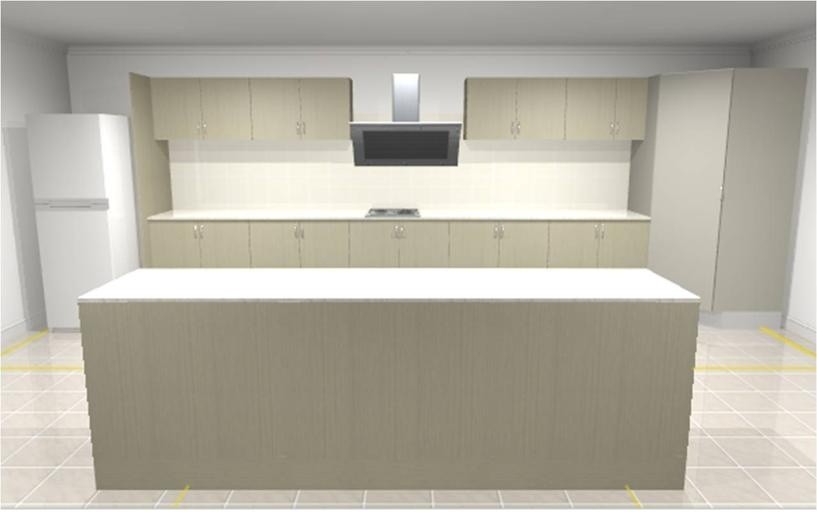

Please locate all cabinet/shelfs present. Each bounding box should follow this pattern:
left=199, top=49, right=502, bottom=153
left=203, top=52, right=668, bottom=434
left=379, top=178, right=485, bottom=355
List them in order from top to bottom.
left=149, top=77, right=251, bottom=143
left=461, top=77, right=566, bottom=143
left=566, top=77, right=650, bottom=142
left=251, top=78, right=354, bottom=143
left=146, top=221, right=249, bottom=268
left=249, top=221, right=350, bottom=267
left=349, top=221, right=450, bottom=268
left=449, top=221, right=548, bottom=268
left=548, top=221, right=650, bottom=268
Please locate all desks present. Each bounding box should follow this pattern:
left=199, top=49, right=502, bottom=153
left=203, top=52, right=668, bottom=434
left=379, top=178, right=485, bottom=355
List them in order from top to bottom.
left=74, top=265, right=704, bottom=492
